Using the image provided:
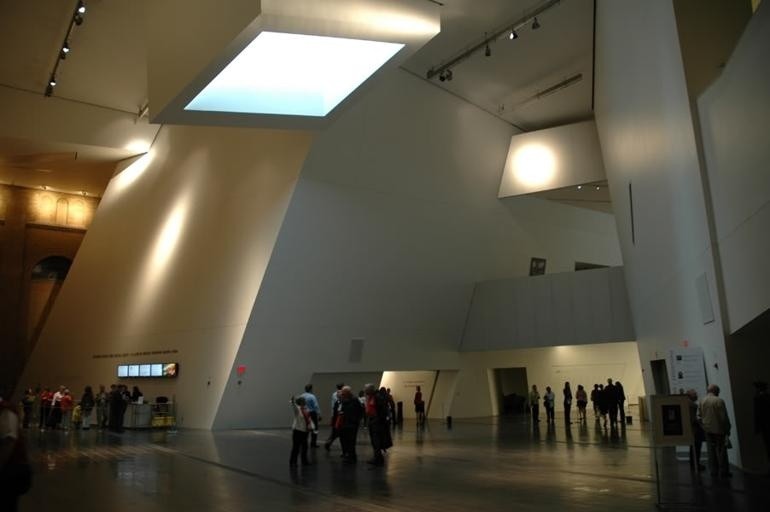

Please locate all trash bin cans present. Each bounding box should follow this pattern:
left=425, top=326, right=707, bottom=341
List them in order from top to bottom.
left=639, top=396, right=649, bottom=422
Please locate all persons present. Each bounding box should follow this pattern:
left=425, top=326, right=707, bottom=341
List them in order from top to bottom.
left=576, top=376, right=626, bottom=426
left=287, top=381, right=395, bottom=466
left=752, top=381, right=769, bottom=464
left=20, top=382, right=143, bottom=436
left=562, top=382, right=573, bottom=424
left=531, top=384, right=541, bottom=422
left=413, top=385, right=422, bottom=425
left=695, top=385, right=734, bottom=485
left=544, top=386, right=555, bottom=424
left=681, top=389, right=709, bottom=471
left=0, top=395, right=20, bottom=512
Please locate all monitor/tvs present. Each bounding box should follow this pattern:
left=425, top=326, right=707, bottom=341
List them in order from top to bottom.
left=117, top=363, right=179, bottom=378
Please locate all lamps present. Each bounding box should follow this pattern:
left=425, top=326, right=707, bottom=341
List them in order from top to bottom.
left=35, top=0, right=91, bottom=102
left=426, top=0, right=562, bottom=88
